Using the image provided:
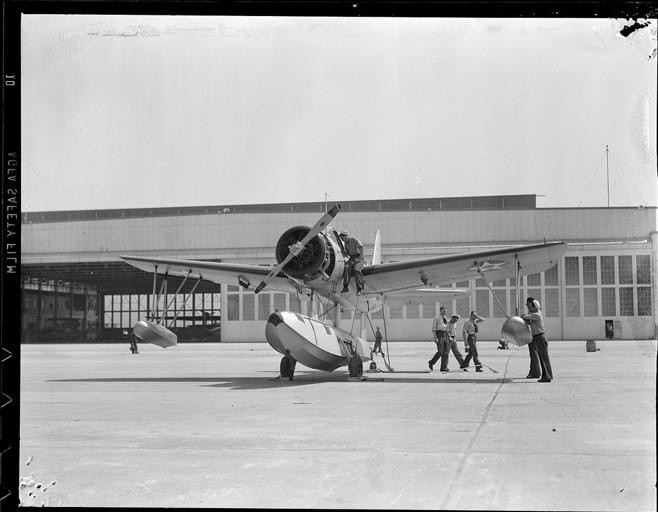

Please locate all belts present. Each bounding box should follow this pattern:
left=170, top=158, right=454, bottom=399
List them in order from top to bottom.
left=437, top=329, right=448, bottom=334
left=535, top=332, right=545, bottom=336
left=468, top=333, right=479, bottom=337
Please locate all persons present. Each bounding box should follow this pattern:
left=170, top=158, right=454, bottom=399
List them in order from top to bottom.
left=340, top=231, right=364, bottom=296
left=520, top=298, right=553, bottom=383
left=428, top=306, right=485, bottom=372
left=606, top=322, right=613, bottom=340
left=373, top=327, right=382, bottom=353
left=497, top=339, right=509, bottom=350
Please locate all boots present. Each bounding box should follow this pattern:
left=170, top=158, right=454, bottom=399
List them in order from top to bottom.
left=341, top=285, right=350, bottom=293
left=356, top=288, right=361, bottom=295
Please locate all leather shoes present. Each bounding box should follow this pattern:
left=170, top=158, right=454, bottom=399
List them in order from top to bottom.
left=428, top=361, right=435, bottom=371
left=440, top=363, right=485, bottom=372
left=527, top=374, right=540, bottom=378
left=537, top=377, right=553, bottom=383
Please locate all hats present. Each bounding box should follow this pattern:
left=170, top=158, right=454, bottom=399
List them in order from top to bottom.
left=340, top=231, right=349, bottom=237
left=527, top=297, right=540, bottom=309
left=451, top=314, right=459, bottom=320
left=442, top=316, right=447, bottom=324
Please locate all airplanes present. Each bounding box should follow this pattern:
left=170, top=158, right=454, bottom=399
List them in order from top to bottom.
left=117, top=203, right=566, bottom=371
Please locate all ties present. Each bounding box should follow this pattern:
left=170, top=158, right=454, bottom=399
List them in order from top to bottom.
left=473, top=321, right=479, bottom=333
